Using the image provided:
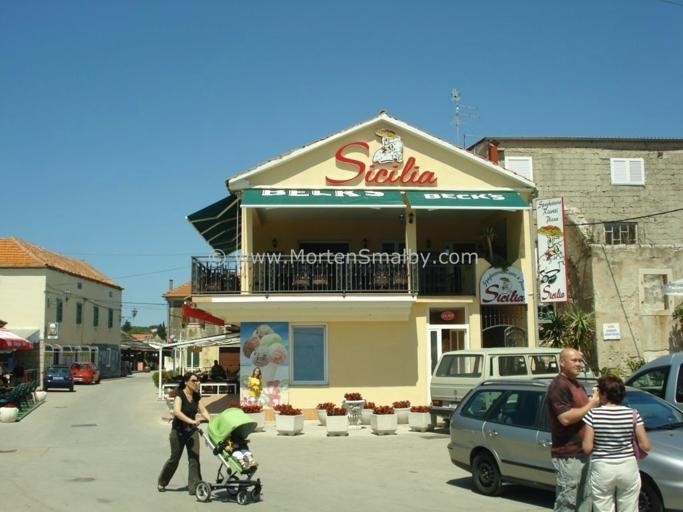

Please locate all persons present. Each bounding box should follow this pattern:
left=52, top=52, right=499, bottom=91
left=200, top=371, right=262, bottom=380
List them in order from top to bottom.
left=546, top=348, right=601, bottom=512
left=12, top=360, right=24, bottom=386
left=210, top=360, right=227, bottom=380
left=0, top=361, right=7, bottom=387
left=227, top=363, right=240, bottom=393
left=248, top=367, right=262, bottom=401
left=158, top=372, right=203, bottom=495
left=582, top=375, right=651, bottom=512
left=224, top=433, right=256, bottom=469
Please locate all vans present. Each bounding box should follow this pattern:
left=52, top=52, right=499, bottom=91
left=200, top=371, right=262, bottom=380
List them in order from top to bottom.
left=428, top=346, right=598, bottom=431
left=623, top=352, right=682, bottom=411
left=447, top=373, right=682, bottom=512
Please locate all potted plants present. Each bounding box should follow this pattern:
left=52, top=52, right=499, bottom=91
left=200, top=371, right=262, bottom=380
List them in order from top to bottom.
left=35, top=388, right=47, bottom=401
left=316, top=392, right=397, bottom=435
left=393, top=400, right=410, bottom=424
left=240, top=402, right=265, bottom=430
left=0, top=403, right=18, bottom=423
left=273, top=404, right=303, bottom=437
left=408, top=405, right=432, bottom=431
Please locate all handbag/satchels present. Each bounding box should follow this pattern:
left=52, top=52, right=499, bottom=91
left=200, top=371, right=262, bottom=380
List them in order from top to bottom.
left=632, top=430, right=647, bottom=461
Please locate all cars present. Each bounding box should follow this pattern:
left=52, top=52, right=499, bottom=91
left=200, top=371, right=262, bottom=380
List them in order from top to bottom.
left=71, top=361, right=100, bottom=385
left=43, top=365, right=73, bottom=391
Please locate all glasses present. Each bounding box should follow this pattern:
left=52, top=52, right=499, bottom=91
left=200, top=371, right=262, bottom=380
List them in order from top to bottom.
left=190, top=380, right=197, bottom=383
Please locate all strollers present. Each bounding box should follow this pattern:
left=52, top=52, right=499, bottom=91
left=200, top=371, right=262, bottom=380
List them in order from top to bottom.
left=191, top=407, right=262, bottom=506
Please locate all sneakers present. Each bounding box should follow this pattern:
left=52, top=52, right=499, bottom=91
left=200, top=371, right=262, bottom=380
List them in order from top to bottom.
left=250, top=460, right=256, bottom=466
left=242, top=463, right=250, bottom=469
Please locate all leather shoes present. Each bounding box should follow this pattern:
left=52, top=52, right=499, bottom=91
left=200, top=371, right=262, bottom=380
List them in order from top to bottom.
left=157, top=483, right=164, bottom=492
